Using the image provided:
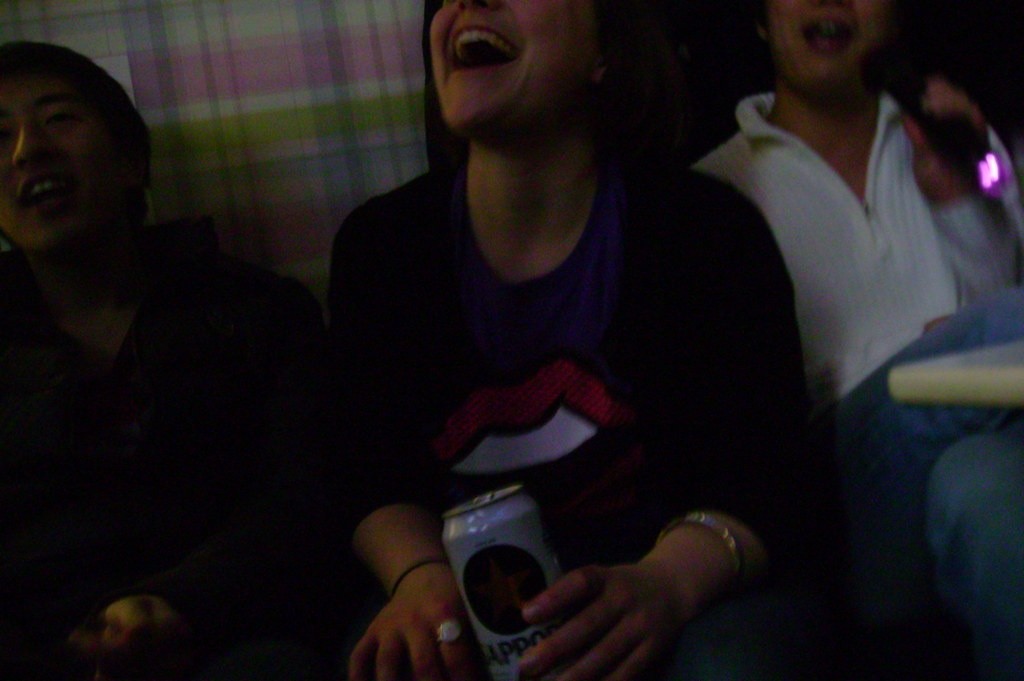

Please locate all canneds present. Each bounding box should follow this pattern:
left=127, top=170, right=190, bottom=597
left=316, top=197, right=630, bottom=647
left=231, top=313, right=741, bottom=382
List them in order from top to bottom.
left=441, top=486, right=564, bottom=681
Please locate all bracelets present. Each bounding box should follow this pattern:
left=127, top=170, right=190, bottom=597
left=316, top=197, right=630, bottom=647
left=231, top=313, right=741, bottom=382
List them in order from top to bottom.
left=655, top=510, right=745, bottom=576
left=391, top=559, right=450, bottom=597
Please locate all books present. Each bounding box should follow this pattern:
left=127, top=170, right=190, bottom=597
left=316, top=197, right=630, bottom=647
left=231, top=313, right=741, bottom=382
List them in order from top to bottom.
left=889, top=339, right=1024, bottom=405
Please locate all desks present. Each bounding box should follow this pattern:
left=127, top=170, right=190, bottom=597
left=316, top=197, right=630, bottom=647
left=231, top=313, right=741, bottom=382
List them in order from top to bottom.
left=888, top=342, right=1024, bottom=414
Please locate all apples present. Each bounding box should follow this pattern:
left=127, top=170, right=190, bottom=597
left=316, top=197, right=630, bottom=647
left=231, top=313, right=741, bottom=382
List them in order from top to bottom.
left=104, top=594, right=186, bottom=652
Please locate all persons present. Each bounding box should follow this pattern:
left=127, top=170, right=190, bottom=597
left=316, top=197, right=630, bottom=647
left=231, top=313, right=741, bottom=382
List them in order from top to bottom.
left=0, top=0, right=1024, bottom=681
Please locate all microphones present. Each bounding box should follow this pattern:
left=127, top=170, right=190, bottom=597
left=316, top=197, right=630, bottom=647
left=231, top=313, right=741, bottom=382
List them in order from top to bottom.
left=857, top=47, right=989, bottom=176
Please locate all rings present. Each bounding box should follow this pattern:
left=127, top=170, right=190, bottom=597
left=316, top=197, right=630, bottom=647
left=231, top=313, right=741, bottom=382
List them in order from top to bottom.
left=436, top=620, right=462, bottom=644
